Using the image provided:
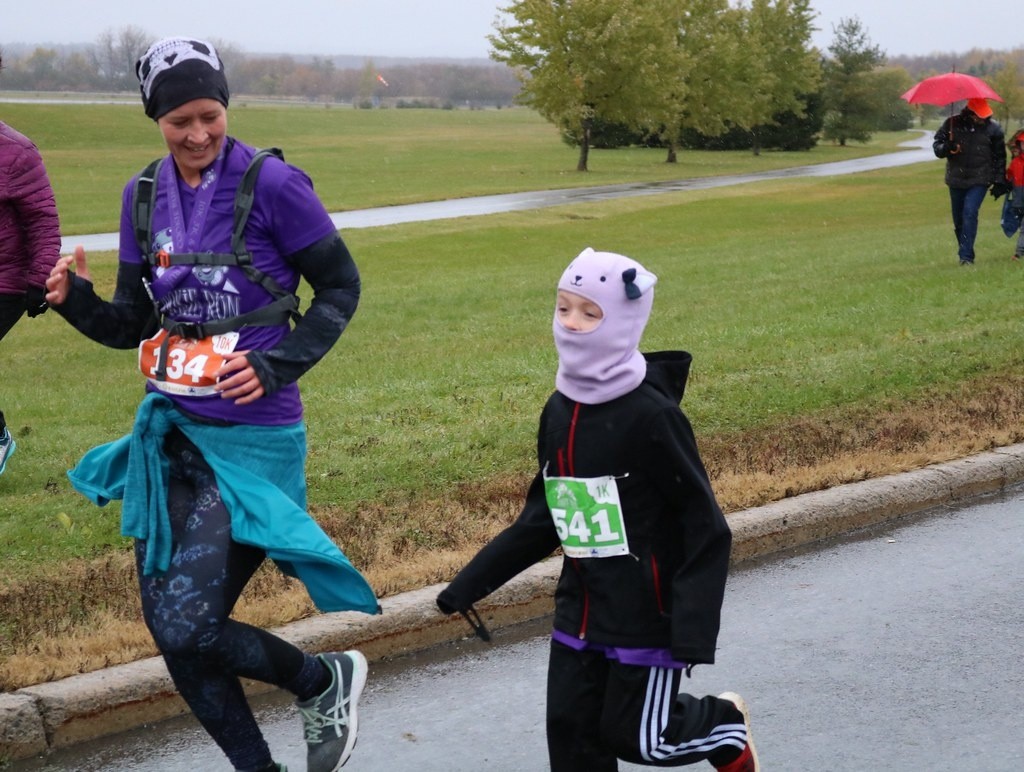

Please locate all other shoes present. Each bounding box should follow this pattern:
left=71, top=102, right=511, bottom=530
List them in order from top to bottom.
left=1012, top=254, right=1021, bottom=262
left=959, top=260, right=972, bottom=267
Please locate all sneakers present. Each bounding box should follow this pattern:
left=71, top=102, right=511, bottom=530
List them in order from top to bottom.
left=0, top=426, right=16, bottom=474
left=293, top=649, right=367, bottom=772
left=716, top=691, right=759, bottom=772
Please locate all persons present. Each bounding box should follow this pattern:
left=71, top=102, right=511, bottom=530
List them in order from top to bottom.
left=0, top=36, right=368, bottom=772
left=933, top=99, right=1024, bottom=265
left=435, top=246, right=761, bottom=772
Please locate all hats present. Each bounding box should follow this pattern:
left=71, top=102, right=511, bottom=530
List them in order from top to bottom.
left=134, top=37, right=230, bottom=124
left=967, top=98, right=994, bottom=118
left=551, top=248, right=660, bottom=404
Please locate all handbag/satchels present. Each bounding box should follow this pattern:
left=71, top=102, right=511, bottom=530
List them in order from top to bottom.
left=1001, top=189, right=1022, bottom=238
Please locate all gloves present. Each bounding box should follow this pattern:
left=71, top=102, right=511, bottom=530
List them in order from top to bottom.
left=944, top=140, right=958, bottom=156
left=989, top=183, right=1006, bottom=200
left=25, top=283, right=51, bottom=318
left=436, top=585, right=471, bottom=615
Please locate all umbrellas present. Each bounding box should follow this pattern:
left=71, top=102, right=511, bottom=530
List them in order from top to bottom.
left=900, top=65, right=1004, bottom=152
